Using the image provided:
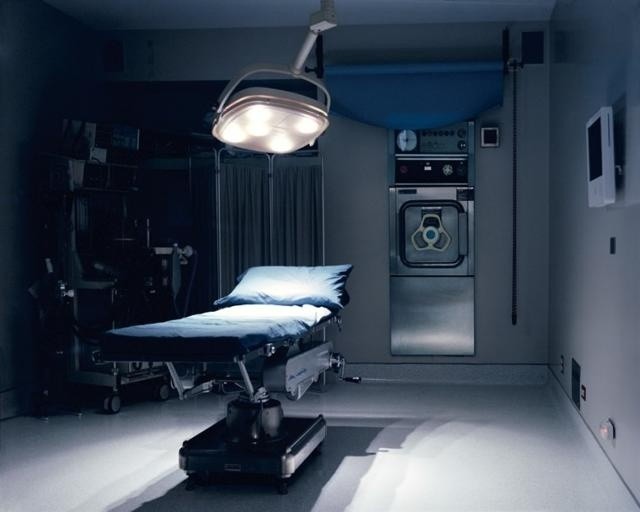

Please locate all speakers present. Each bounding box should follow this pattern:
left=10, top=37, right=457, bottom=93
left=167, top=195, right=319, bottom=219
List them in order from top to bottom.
left=521, top=31, right=545, bottom=64
left=102, top=39, right=124, bottom=72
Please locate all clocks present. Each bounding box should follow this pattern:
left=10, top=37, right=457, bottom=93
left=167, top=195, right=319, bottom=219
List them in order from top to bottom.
left=393, top=129, right=420, bottom=153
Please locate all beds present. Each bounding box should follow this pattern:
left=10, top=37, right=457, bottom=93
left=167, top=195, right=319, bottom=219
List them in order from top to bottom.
left=100, top=282, right=354, bottom=496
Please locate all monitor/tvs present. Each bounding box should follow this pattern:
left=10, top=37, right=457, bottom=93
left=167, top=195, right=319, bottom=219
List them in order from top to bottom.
left=585, top=106, right=616, bottom=208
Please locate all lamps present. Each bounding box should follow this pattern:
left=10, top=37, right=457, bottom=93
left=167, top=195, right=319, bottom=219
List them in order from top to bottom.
left=208, top=1, right=339, bottom=156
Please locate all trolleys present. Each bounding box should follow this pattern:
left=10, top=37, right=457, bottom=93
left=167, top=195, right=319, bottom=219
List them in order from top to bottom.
left=32, top=216, right=199, bottom=414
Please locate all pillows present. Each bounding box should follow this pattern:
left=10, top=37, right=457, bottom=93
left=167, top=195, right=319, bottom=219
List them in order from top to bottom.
left=214, top=262, right=355, bottom=311
